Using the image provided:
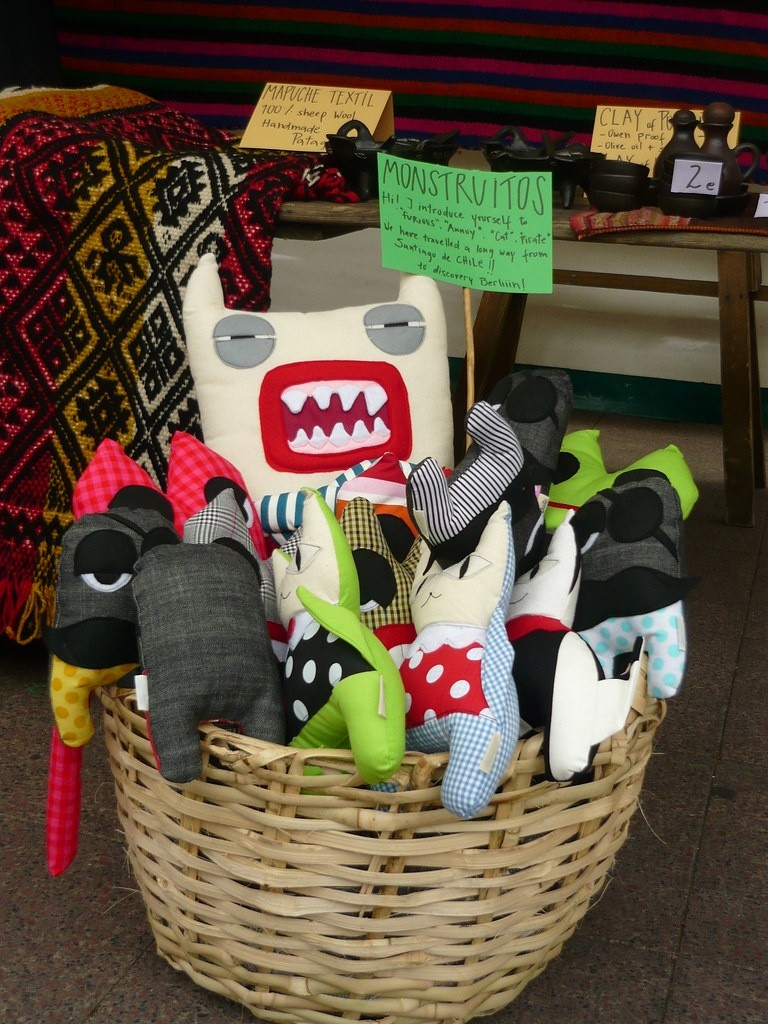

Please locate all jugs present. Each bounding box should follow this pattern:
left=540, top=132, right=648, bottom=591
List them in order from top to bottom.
left=696, top=102, right=759, bottom=193
left=652, top=110, right=701, bottom=194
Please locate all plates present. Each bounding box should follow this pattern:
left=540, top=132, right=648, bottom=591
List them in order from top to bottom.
left=650, top=185, right=750, bottom=220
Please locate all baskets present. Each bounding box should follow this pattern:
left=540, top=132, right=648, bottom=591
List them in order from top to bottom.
left=94, top=651, right=668, bottom=1024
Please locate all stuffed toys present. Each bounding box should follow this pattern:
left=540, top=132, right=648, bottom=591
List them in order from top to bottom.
left=45, top=252, right=702, bottom=875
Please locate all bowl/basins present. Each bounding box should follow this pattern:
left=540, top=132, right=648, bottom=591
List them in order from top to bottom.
left=592, top=160, right=649, bottom=194
left=589, top=190, right=643, bottom=213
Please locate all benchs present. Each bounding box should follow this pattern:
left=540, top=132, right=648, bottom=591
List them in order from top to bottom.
left=278, top=201, right=768, bottom=528
